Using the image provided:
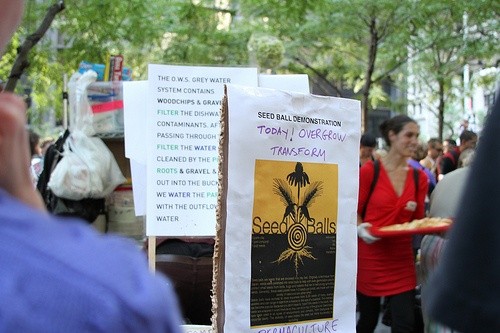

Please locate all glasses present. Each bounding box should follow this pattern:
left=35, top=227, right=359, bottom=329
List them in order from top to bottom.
left=432, top=146, right=444, bottom=152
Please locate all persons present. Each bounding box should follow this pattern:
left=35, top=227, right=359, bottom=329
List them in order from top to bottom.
left=0, top=0, right=185, bottom=333
left=357, top=79, right=500, bottom=333
